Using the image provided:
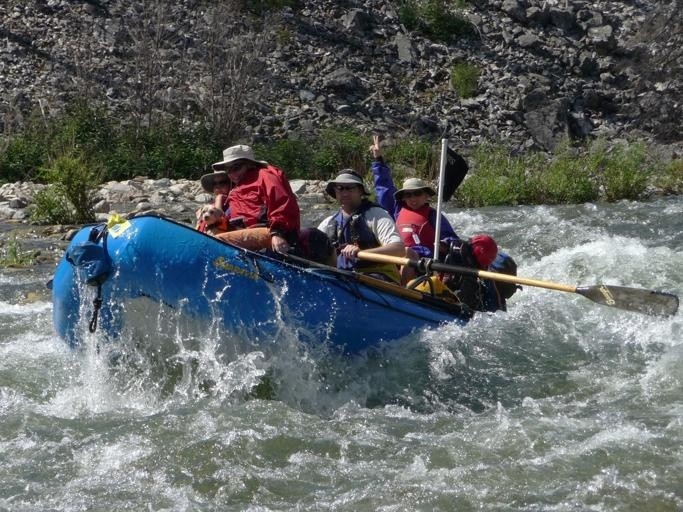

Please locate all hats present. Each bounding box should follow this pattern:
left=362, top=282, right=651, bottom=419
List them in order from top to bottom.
left=212, top=144, right=269, bottom=171
left=325, top=168, right=371, bottom=200
left=200, top=170, right=228, bottom=193
left=393, top=178, right=436, bottom=202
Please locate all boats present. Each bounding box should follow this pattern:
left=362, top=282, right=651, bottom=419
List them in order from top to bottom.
left=53, top=216, right=471, bottom=383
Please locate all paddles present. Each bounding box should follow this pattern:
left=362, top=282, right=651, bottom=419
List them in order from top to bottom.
left=355, top=251, right=679, bottom=319
left=289, top=252, right=474, bottom=322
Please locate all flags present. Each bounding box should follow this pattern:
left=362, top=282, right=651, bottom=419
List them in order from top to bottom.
left=435, top=146, right=468, bottom=202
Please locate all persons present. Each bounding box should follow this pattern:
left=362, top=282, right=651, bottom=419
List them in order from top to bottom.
left=367, top=134, right=462, bottom=287
left=210, top=143, right=302, bottom=265
left=195, top=167, right=236, bottom=233
left=317, top=167, right=406, bottom=290
